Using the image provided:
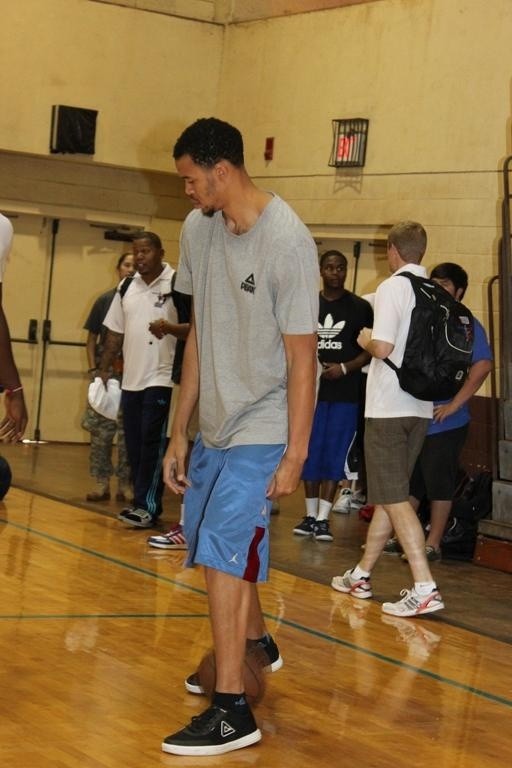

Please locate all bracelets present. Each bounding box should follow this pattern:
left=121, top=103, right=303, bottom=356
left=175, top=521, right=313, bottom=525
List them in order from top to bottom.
left=339, top=361, right=347, bottom=376
left=5, top=385, right=24, bottom=396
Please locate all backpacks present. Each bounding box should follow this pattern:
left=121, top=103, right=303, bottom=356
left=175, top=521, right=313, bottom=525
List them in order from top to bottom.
left=383, top=269, right=476, bottom=402
left=440, top=467, right=493, bottom=560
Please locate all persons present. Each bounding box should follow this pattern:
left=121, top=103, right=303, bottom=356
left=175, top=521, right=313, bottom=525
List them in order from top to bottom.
left=161, top=116, right=322, bottom=754
left=1, top=209, right=29, bottom=446
left=330, top=220, right=445, bottom=618
left=82, top=230, right=192, bottom=551
left=293, top=250, right=374, bottom=543
left=362, top=262, right=494, bottom=565
left=331, top=292, right=375, bottom=515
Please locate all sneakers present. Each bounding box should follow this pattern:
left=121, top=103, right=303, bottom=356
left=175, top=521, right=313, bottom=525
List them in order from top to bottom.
left=328, top=486, right=444, bottom=565
left=85, top=488, right=111, bottom=501
left=118, top=506, right=135, bottom=520
left=123, top=507, right=157, bottom=526
left=314, top=514, right=333, bottom=539
left=293, top=515, right=316, bottom=535
left=149, top=520, right=188, bottom=549
left=332, top=567, right=373, bottom=599
left=381, top=586, right=444, bottom=617
left=185, top=633, right=284, bottom=694
left=161, top=700, right=262, bottom=756
